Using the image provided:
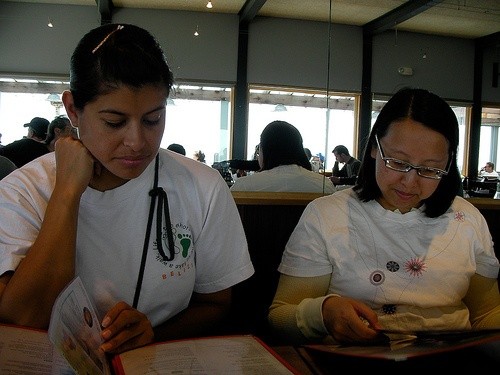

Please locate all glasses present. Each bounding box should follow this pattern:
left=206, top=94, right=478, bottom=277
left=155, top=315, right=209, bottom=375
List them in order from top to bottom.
left=375, top=134, right=453, bottom=179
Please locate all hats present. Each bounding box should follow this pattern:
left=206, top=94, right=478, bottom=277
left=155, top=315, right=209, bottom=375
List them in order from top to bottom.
left=24, top=117, right=51, bottom=134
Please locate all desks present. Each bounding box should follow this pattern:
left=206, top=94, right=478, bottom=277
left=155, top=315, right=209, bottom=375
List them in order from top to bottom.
left=269, top=346, right=500, bottom=375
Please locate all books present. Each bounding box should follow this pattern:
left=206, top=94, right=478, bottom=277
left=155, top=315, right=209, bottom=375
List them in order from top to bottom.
left=0, top=273, right=302, bottom=374
left=303, top=315, right=499, bottom=360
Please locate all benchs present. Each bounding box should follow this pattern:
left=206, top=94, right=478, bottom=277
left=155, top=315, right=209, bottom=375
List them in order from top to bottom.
left=230, top=191, right=500, bottom=317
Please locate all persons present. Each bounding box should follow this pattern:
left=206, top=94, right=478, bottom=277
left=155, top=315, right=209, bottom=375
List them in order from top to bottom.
left=0, top=22, right=256, bottom=355
left=267, top=85, right=499, bottom=344
left=0, top=114, right=79, bottom=168
left=229, top=120, right=337, bottom=193
left=319, top=145, right=362, bottom=185
left=479, top=161, right=500, bottom=176
left=478, top=162, right=499, bottom=177
